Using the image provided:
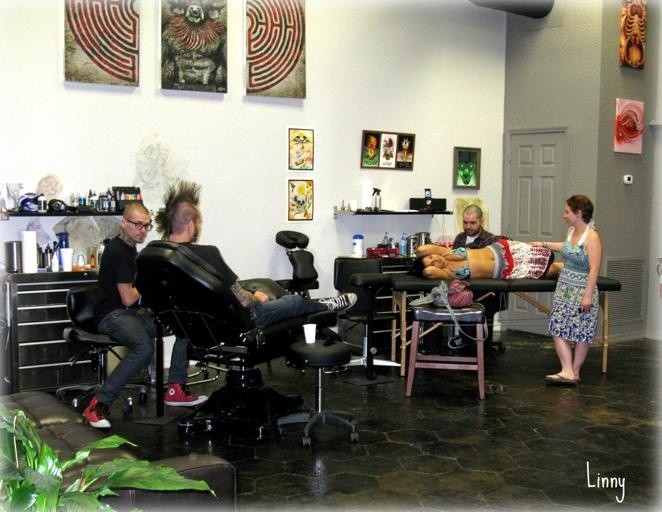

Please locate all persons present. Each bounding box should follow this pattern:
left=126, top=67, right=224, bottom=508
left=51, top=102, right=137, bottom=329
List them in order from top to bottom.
left=156, top=180, right=358, bottom=326
left=527, top=193, right=602, bottom=384
left=81, top=201, right=210, bottom=429
left=454, top=204, right=496, bottom=248
left=409, top=239, right=564, bottom=279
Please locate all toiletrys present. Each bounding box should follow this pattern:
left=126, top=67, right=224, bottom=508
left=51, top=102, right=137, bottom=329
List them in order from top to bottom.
left=71, top=189, right=115, bottom=212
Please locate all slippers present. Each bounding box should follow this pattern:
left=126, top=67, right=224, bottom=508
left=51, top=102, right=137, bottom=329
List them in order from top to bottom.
left=544, top=373, right=582, bottom=384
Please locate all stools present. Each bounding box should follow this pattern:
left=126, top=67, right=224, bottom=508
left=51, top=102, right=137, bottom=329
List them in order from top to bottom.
left=405, top=303, right=486, bottom=400
left=275, top=340, right=359, bottom=450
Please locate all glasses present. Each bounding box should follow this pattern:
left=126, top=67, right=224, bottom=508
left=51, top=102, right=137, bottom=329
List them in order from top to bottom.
left=123, top=217, right=153, bottom=232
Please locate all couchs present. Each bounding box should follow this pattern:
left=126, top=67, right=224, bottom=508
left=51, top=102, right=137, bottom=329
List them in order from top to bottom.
left=0, top=390, right=239, bottom=512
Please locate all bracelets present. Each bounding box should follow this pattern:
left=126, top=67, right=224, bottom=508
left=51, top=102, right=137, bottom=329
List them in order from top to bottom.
left=542, top=241, right=547, bottom=248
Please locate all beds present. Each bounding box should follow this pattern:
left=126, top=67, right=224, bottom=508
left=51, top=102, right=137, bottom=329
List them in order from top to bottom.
left=384, top=273, right=621, bottom=377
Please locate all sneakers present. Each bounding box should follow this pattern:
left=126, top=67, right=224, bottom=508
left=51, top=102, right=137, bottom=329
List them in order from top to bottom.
left=318, top=293, right=358, bottom=312
left=163, top=383, right=209, bottom=408
left=82, top=395, right=112, bottom=430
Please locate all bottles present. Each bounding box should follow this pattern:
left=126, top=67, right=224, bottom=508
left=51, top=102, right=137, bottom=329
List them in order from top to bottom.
left=70, top=187, right=117, bottom=214
left=36, top=194, right=46, bottom=213
left=5, top=230, right=105, bottom=274
left=352, top=234, right=364, bottom=256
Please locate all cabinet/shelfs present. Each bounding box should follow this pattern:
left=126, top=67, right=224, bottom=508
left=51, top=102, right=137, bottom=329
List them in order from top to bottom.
left=0, top=270, right=100, bottom=393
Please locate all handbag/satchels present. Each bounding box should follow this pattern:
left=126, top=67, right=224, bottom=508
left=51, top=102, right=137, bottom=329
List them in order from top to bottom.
left=408, top=279, right=449, bottom=308
left=449, top=279, right=475, bottom=309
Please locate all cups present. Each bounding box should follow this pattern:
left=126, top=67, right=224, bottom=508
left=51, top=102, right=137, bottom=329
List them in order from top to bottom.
left=302, top=323, right=318, bottom=344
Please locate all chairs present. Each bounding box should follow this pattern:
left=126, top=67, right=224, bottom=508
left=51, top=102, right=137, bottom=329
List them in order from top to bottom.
left=275, top=230, right=319, bottom=301
left=132, top=238, right=304, bottom=448
left=334, top=256, right=400, bottom=387
left=55, top=285, right=148, bottom=416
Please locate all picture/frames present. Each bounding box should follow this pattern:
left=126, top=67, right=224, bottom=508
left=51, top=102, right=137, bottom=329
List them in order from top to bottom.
left=360, top=129, right=415, bottom=170
left=453, top=147, right=481, bottom=189
